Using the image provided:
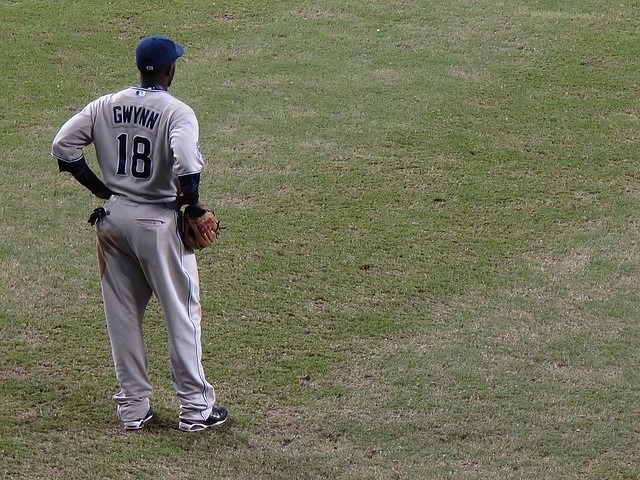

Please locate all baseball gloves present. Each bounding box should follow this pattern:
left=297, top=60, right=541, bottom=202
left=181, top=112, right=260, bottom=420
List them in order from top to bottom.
left=183, top=206, right=226, bottom=250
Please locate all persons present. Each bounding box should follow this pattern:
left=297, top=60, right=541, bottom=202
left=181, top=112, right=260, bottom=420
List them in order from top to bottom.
left=50, top=36, right=228, bottom=431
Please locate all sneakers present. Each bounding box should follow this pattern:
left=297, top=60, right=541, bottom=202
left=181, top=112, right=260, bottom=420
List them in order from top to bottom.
left=123, top=407, right=153, bottom=430
left=178, top=407, right=228, bottom=432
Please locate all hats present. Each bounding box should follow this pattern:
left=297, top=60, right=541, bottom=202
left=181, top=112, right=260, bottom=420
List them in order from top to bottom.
left=136, top=35, right=184, bottom=72
